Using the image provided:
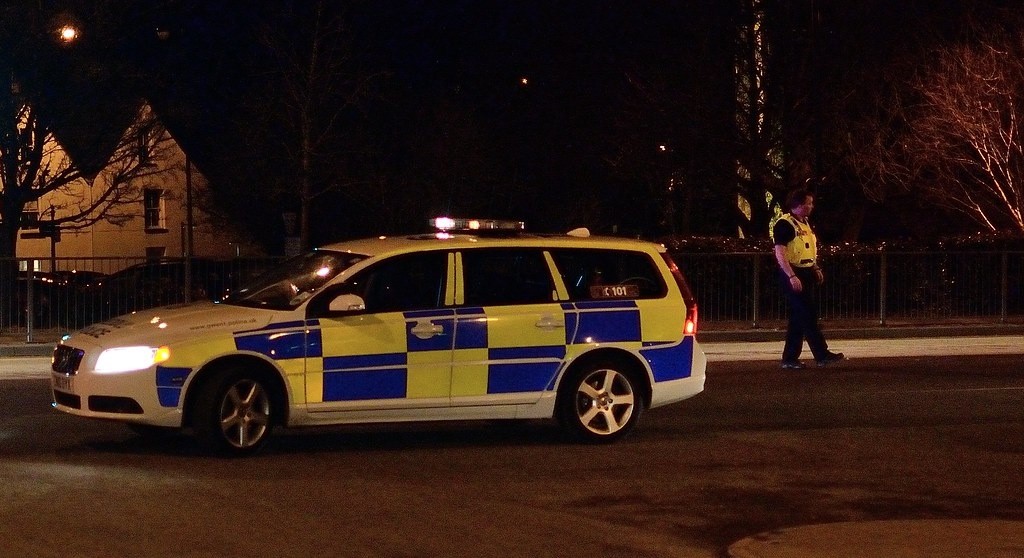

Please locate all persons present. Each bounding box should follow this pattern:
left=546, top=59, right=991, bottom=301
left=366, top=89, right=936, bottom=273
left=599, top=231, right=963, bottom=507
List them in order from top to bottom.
left=773, top=191, right=844, bottom=369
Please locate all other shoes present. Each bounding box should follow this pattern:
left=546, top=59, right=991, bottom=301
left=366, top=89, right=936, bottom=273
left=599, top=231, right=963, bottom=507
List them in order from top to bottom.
left=817, top=353, right=845, bottom=368
left=780, top=359, right=804, bottom=369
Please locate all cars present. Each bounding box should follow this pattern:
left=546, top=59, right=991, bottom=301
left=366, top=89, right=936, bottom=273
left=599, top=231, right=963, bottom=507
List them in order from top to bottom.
left=51, top=216, right=707, bottom=460
left=16, top=258, right=282, bottom=328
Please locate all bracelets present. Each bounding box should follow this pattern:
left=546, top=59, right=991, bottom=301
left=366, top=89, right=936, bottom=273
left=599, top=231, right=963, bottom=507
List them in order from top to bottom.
left=815, top=268, right=820, bottom=271
left=790, top=275, right=796, bottom=278
